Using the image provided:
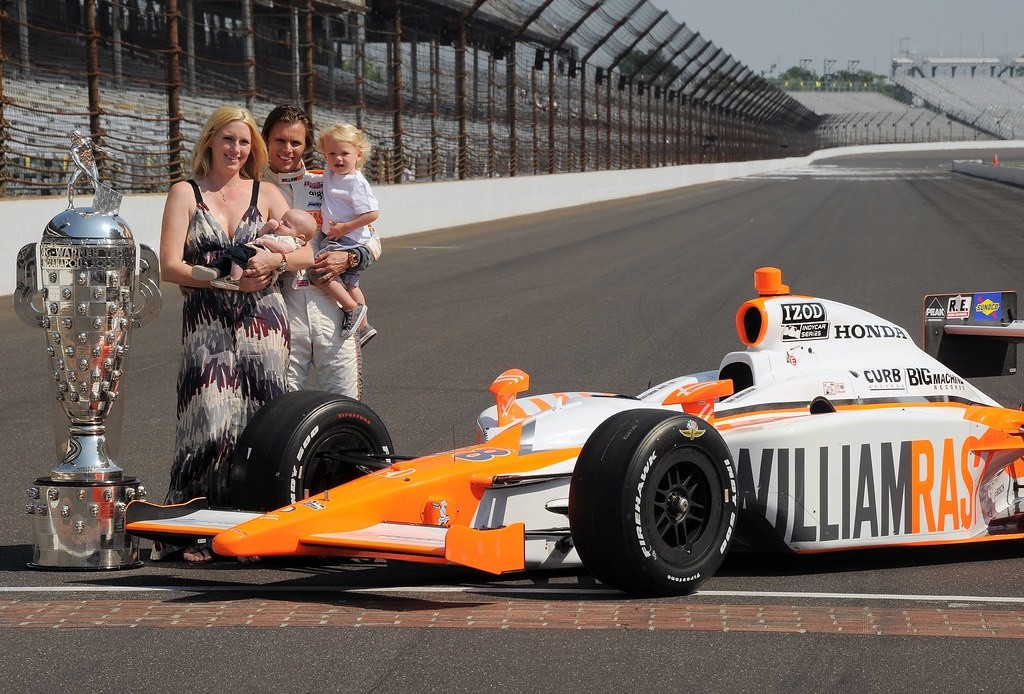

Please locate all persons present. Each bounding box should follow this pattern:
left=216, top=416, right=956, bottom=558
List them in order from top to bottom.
left=261, top=104, right=382, bottom=405
left=159, top=106, right=314, bottom=562
left=307, top=123, right=378, bottom=348
left=190, top=209, right=317, bottom=290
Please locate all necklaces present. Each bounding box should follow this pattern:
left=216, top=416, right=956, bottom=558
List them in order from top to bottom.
left=207, top=177, right=241, bottom=202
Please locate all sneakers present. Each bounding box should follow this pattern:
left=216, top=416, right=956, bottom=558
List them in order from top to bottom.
left=340, top=303, right=368, bottom=339
left=359, top=324, right=377, bottom=348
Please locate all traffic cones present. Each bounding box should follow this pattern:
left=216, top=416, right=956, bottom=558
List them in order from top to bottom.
left=992, top=153, right=999, bottom=167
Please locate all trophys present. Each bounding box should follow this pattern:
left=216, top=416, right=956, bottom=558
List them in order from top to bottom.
left=13, top=129, right=163, bottom=570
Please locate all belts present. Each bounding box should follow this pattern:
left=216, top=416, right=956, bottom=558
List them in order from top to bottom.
left=277, top=279, right=317, bottom=286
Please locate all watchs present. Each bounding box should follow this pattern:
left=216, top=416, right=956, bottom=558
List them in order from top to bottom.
left=346, top=249, right=359, bottom=272
left=276, top=252, right=288, bottom=272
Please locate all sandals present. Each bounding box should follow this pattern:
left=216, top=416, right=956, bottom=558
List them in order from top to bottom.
left=183, top=547, right=213, bottom=564
left=236, top=561, right=261, bottom=567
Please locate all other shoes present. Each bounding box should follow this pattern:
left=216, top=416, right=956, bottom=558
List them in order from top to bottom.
left=191, top=265, right=217, bottom=281
left=211, top=275, right=240, bottom=291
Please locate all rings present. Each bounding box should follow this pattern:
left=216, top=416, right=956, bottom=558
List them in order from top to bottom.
left=326, top=274, right=331, bottom=279
